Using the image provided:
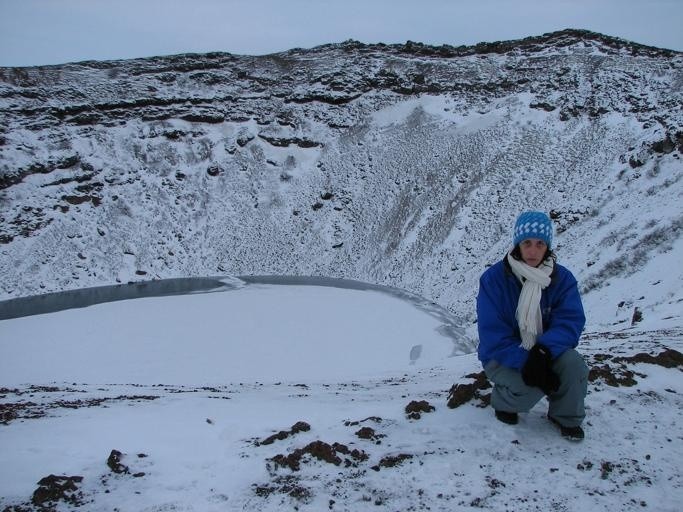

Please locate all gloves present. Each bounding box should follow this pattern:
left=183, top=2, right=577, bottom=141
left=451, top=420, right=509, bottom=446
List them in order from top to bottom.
left=522, top=343, right=561, bottom=394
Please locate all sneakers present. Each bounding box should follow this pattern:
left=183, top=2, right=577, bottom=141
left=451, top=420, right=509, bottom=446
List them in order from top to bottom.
left=495, top=411, right=518, bottom=425
left=547, top=415, right=584, bottom=441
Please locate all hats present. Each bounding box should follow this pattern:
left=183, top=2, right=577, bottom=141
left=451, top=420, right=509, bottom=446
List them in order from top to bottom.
left=513, top=211, right=553, bottom=250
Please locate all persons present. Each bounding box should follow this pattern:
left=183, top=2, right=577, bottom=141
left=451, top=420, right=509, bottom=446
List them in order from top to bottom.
left=476, top=211, right=589, bottom=441
